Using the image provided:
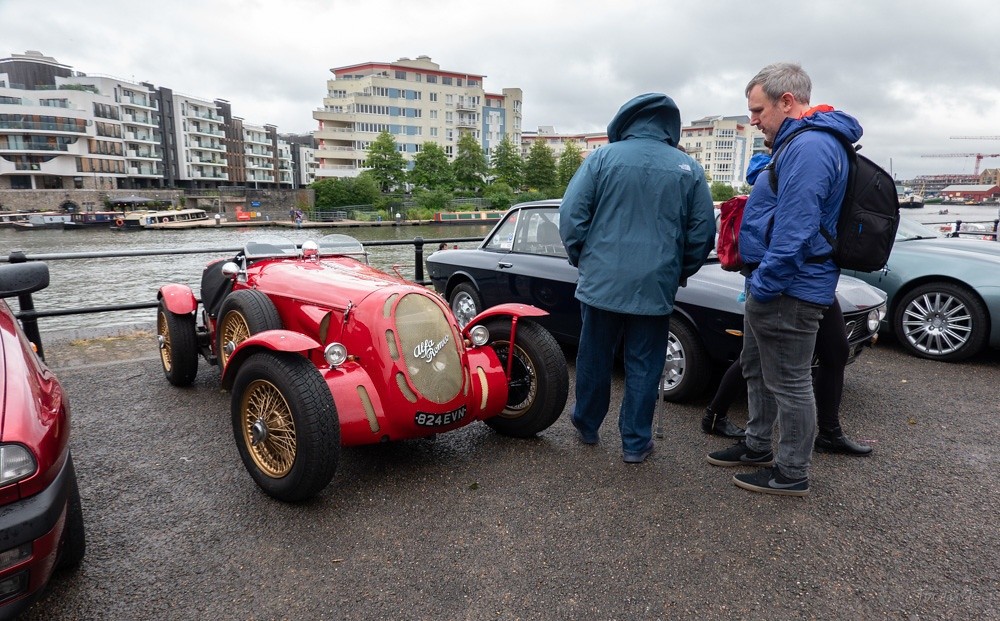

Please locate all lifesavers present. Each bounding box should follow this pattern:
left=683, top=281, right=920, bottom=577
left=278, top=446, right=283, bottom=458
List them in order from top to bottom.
left=163, top=218, right=169, bottom=223
left=116, top=219, right=124, bottom=226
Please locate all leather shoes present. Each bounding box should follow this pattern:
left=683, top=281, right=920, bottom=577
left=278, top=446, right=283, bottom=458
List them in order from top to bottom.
left=701, top=406, right=746, bottom=440
left=814, top=426, right=873, bottom=456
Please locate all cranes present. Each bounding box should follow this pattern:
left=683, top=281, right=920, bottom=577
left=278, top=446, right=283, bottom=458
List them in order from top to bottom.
left=921, top=134, right=1000, bottom=175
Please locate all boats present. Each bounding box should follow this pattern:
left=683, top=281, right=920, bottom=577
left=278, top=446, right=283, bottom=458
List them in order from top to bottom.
left=898, top=195, right=925, bottom=208
left=108, top=209, right=209, bottom=231
left=0, top=211, right=28, bottom=227
left=63, top=212, right=124, bottom=230
left=12, top=213, right=72, bottom=231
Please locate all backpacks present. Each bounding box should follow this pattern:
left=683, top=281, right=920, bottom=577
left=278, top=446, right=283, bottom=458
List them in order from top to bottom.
left=768, top=125, right=900, bottom=283
left=717, top=194, right=749, bottom=272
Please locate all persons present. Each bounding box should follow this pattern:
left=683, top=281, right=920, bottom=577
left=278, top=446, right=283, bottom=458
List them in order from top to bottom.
left=706, top=61, right=863, bottom=497
left=558, top=92, right=716, bottom=463
left=289, top=207, right=303, bottom=225
left=435, top=243, right=448, bottom=253
left=701, top=270, right=873, bottom=456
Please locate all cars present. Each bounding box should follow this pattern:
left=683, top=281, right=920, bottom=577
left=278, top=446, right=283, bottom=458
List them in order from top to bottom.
left=424, top=199, right=890, bottom=404
left=712, top=201, right=1000, bottom=359
left=154, top=233, right=571, bottom=502
left=0, top=260, right=84, bottom=616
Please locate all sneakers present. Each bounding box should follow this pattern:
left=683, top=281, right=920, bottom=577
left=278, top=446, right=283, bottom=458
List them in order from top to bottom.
left=571, top=404, right=599, bottom=444
left=707, top=437, right=775, bottom=467
left=623, top=440, right=654, bottom=463
left=732, top=467, right=810, bottom=496
